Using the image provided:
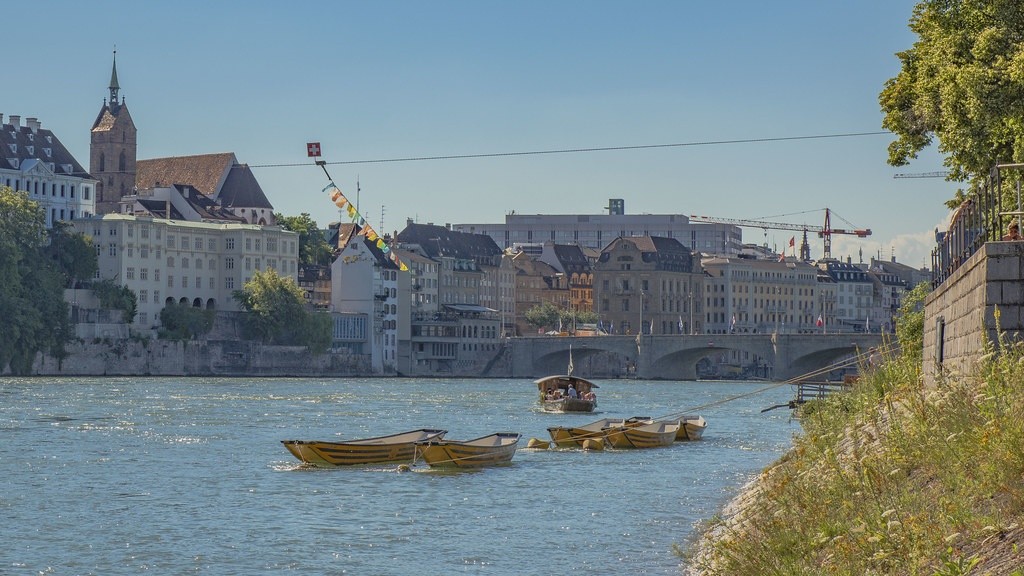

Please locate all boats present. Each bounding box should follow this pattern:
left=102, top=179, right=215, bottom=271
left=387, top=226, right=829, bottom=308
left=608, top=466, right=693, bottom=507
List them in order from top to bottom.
left=532, top=345, right=600, bottom=414
left=673, top=415, right=708, bottom=440
left=546, top=417, right=626, bottom=450
left=600, top=419, right=682, bottom=451
left=279, top=427, right=449, bottom=468
left=413, top=431, right=522, bottom=471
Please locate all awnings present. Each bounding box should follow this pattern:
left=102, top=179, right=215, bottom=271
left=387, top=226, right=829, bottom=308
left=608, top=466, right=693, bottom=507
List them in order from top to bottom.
left=442, top=303, right=500, bottom=313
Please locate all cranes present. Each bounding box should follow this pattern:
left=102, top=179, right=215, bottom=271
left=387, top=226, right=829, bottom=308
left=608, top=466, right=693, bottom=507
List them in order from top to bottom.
left=688, top=207, right=873, bottom=266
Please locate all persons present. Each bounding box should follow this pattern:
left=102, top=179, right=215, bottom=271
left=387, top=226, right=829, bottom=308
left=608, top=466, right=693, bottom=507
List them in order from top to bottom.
left=546, top=384, right=595, bottom=400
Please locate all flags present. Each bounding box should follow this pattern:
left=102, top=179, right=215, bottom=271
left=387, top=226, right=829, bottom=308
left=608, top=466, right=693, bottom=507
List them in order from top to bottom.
left=321, top=181, right=409, bottom=272
left=789, top=237, right=795, bottom=247
left=778, top=250, right=785, bottom=262
left=554, top=314, right=870, bottom=334
